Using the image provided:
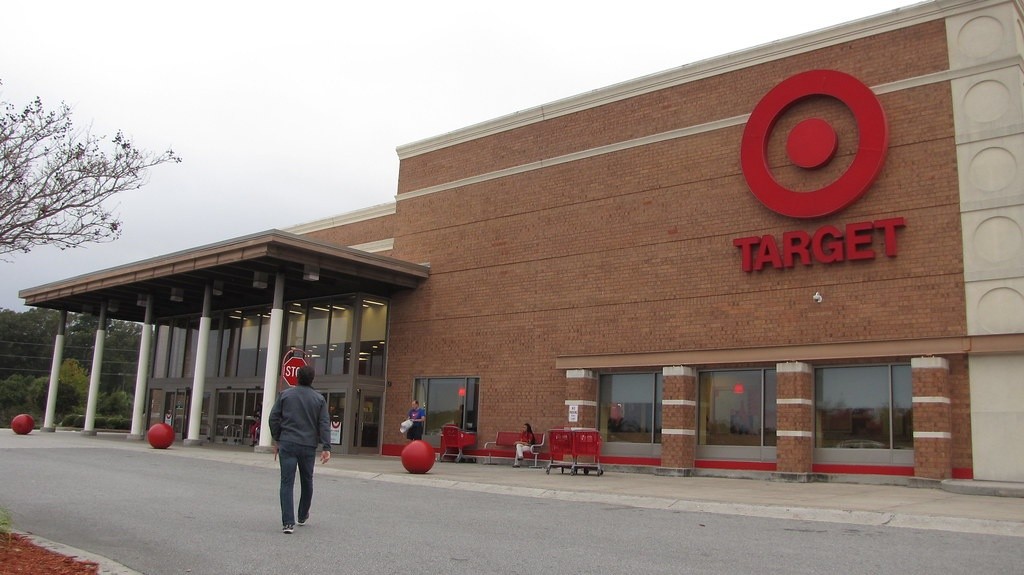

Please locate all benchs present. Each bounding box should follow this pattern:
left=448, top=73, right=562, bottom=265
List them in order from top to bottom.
left=484, top=431, right=545, bottom=468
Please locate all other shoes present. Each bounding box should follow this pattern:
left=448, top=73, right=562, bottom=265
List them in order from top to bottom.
left=518, top=458, right=523, bottom=461
left=512, top=465, right=520, bottom=468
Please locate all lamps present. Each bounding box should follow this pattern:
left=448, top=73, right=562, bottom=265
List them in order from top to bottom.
left=734, top=383, right=744, bottom=394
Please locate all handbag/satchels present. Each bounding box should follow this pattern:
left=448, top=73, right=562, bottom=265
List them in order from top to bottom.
left=527, top=432, right=535, bottom=445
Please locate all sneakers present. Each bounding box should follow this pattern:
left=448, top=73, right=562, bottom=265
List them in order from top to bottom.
left=298, top=512, right=309, bottom=525
left=282, top=524, right=294, bottom=533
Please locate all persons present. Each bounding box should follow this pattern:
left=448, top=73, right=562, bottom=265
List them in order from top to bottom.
left=268, top=366, right=331, bottom=534
left=407, top=400, right=426, bottom=441
left=250, top=415, right=261, bottom=446
left=512, top=423, right=535, bottom=467
